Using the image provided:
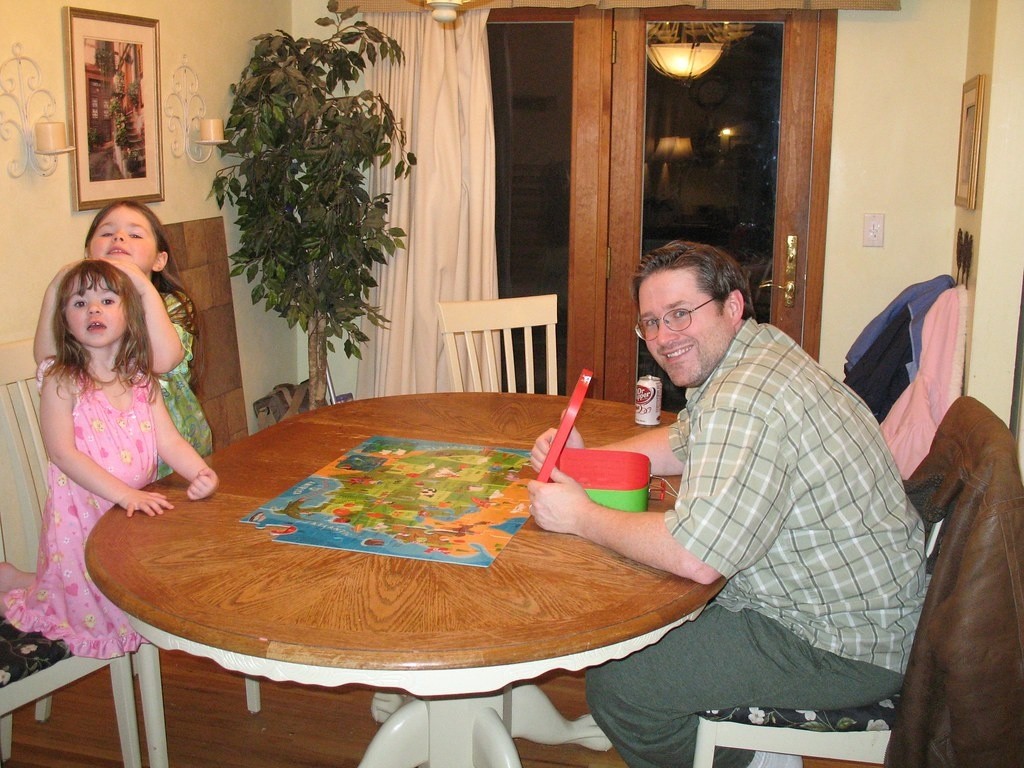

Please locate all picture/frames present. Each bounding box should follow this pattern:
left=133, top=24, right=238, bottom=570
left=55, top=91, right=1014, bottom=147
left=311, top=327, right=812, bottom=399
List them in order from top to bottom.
left=65, top=1, right=168, bottom=210
left=951, top=71, right=986, bottom=211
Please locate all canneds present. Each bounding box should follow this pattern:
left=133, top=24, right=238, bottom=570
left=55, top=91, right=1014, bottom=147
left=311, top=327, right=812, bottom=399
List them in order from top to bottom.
left=635, top=375, right=662, bottom=426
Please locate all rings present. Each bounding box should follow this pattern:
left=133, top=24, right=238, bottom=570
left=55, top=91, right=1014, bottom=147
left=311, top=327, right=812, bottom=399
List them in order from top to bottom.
left=528, top=504, right=533, bottom=512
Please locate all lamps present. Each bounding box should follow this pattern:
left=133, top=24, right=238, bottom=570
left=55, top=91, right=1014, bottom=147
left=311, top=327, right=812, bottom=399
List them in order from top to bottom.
left=646, top=18, right=758, bottom=87
left=2, top=42, right=77, bottom=177
left=164, top=59, right=227, bottom=164
left=645, top=134, right=697, bottom=224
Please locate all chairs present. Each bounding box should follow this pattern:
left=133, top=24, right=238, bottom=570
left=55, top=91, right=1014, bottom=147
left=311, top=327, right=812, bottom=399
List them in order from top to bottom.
left=833, top=272, right=970, bottom=484
left=436, top=295, right=560, bottom=402
left=0, top=330, right=262, bottom=768
left=694, top=394, right=1024, bottom=768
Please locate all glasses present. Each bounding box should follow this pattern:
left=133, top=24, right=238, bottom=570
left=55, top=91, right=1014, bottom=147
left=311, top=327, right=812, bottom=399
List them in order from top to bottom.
left=633, top=287, right=746, bottom=341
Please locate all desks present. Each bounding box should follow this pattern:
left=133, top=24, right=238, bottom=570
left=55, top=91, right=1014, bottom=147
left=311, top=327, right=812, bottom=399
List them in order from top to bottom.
left=87, top=393, right=727, bottom=768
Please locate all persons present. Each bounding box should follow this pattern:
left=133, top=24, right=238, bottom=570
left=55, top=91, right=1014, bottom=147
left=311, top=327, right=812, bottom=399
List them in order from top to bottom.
left=31, top=197, right=215, bottom=481
left=525, top=236, right=929, bottom=768
left=1, top=260, right=219, bottom=664
